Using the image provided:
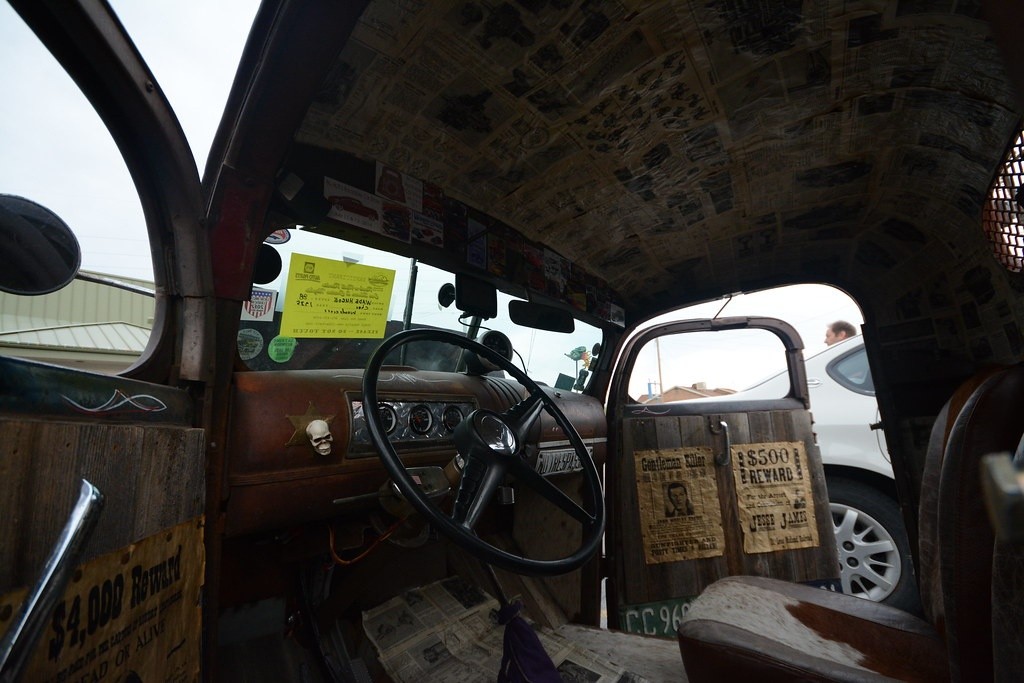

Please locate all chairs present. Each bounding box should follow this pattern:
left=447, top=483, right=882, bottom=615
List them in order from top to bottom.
left=679, top=364, right=1024, bottom=683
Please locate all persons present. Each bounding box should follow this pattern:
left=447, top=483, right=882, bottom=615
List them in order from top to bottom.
left=824, top=322, right=855, bottom=345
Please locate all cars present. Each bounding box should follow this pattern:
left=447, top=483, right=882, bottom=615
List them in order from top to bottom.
left=663, top=333, right=918, bottom=608
